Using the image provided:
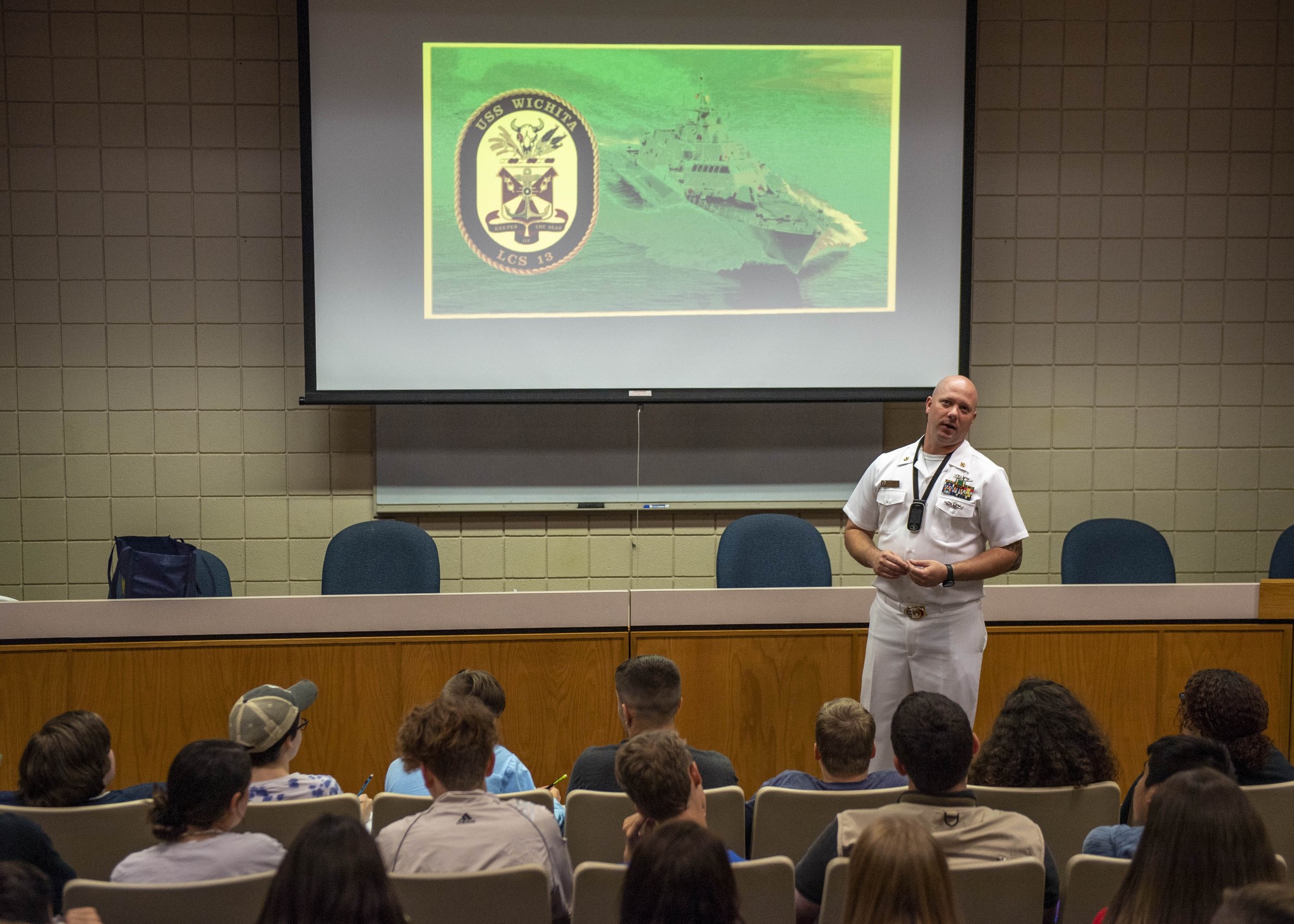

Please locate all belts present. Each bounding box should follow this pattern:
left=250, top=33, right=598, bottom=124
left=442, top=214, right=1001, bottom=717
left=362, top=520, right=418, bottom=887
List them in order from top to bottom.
left=875, top=588, right=978, bottom=619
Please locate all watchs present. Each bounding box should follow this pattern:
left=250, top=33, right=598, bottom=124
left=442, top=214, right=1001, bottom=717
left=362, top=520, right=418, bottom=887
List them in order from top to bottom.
left=942, top=564, right=955, bottom=588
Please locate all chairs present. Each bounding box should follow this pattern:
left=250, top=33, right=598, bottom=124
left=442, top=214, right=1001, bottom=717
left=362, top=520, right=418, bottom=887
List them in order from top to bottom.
left=716, top=514, right=832, bottom=588
left=1061, top=518, right=1176, bottom=584
left=322, top=520, right=440, bottom=594
left=108, top=549, right=232, bottom=598
left=0, top=783, right=1294, bottom=924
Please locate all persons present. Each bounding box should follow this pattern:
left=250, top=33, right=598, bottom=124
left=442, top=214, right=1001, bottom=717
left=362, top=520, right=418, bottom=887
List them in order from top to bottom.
left=842, top=374, right=1029, bottom=773
left=569, top=653, right=740, bottom=793
left=384, top=668, right=564, bottom=829
left=1120, top=668, right=1294, bottom=824
left=969, top=677, right=1119, bottom=789
left=228, top=681, right=373, bottom=834
left=794, top=691, right=1060, bottom=924
left=374, top=694, right=574, bottom=919
left=746, top=696, right=908, bottom=819
left=20, top=709, right=132, bottom=808
left=615, top=727, right=743, bottom=864
left=1081, top=737, right=1236, bottom=860
left=109, top=740, right=289, bottom=883
left=844, top=818, right=955, bottom=924
left=621, top=819, right=741, bottom=923
left=0, top=857, right=103, bottom=923
left=1211, top=883, right=1294, bottom=924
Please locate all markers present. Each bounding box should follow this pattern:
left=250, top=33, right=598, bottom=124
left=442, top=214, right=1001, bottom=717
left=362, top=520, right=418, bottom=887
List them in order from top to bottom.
left=644, top=505, right=669, bottom=508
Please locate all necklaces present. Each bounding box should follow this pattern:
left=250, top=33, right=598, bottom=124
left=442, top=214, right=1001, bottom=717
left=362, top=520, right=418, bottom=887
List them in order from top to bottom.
left=1091, top=769, right=1273, bottom=924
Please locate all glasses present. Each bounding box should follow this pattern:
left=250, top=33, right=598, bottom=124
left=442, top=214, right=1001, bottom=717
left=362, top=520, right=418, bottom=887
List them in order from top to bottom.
left=1178, top=692, right=1186, bottom=702
left=297, top=716, right=309, bottom=732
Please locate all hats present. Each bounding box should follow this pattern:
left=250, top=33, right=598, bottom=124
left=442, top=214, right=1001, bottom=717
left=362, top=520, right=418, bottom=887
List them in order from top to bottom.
left=228, top=680, right=318, bottom=753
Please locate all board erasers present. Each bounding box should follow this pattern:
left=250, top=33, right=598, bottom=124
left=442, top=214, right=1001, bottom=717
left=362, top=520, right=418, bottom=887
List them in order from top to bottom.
left=578, top=503, right=605, bottom=509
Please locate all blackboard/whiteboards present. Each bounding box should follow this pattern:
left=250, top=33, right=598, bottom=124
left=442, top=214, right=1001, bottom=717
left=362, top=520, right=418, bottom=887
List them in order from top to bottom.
left=370, top=402, right=886, bottom=512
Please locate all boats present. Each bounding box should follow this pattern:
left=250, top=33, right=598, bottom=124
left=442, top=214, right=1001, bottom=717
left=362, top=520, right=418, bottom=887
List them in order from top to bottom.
left=600, top=72, right=862, bottom=287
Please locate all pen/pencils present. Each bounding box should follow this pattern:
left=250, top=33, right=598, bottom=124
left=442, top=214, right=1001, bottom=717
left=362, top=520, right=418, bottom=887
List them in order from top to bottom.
left=357, top=774, right=374, bottom=797
left=546, top=774, right=567, bottom=789
